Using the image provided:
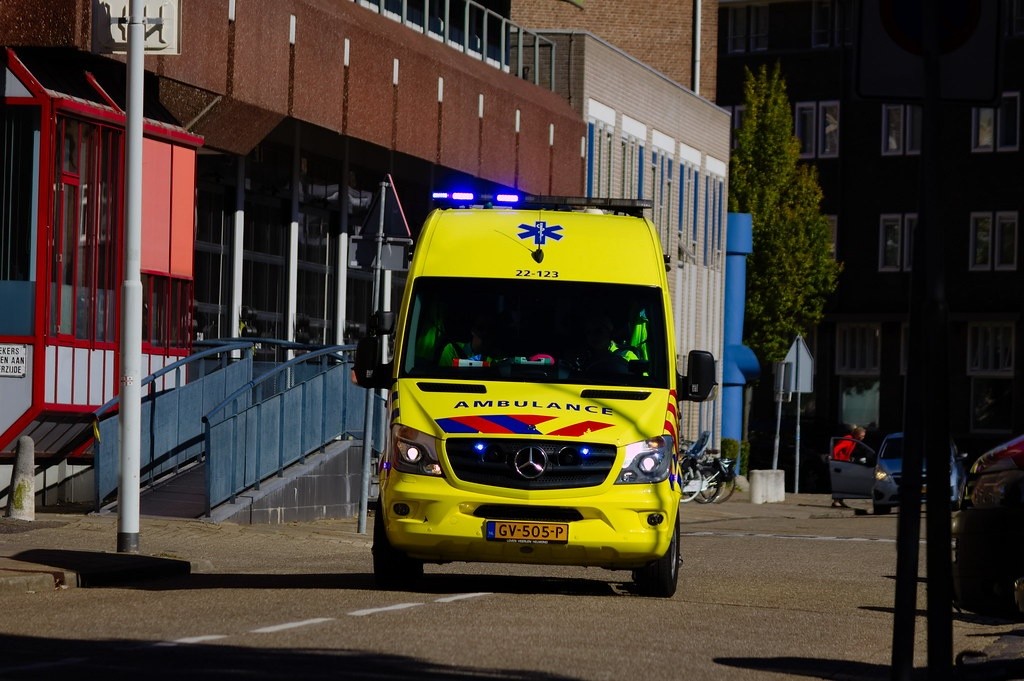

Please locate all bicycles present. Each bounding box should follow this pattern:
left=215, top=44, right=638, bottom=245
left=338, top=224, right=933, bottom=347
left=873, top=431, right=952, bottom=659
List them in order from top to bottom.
left=679, top=438, right=737, bottom=504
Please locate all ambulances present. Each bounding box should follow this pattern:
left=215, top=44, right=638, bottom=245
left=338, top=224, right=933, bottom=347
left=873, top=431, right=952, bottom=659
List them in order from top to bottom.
left=350, top=190, right=718, bottom=598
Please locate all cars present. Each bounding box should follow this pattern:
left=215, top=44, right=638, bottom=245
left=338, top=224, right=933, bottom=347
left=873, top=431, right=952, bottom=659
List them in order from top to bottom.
left=828, top=432, right=968, bottom=515
left=951, top=433, right=1024, bottom=606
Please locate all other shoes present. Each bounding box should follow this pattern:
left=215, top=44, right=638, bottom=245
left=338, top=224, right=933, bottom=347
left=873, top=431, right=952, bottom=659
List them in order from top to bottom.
left=832, top=501, right=850, bottom=508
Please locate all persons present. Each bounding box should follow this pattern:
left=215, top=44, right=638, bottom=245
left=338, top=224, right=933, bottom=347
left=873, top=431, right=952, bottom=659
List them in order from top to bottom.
left=576, top=309, right=639, bottom=376
left=438, top=311, right=503, bottom=373
left=829, top=425, right=866, bottom=508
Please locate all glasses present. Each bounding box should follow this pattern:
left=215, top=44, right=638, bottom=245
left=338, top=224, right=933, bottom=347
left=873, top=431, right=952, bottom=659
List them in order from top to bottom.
left=587, top=328, right=610, bottom=334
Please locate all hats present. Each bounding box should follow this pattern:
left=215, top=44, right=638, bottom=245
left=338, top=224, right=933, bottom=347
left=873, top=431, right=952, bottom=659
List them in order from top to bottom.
left=586, top=313, right=614, bottom=330
